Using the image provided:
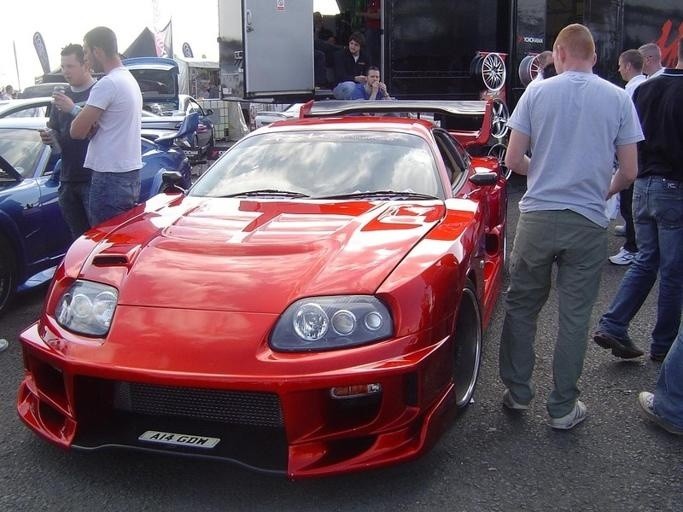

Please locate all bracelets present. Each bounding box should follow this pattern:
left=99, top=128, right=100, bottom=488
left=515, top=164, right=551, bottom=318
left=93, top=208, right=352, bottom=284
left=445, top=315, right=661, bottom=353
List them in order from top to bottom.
left=70, top=104, right=82, bottom=117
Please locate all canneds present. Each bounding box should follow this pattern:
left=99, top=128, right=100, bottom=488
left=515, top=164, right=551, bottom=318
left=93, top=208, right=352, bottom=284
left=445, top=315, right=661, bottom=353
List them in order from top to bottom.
left=54, top=86, right=66, bottom=111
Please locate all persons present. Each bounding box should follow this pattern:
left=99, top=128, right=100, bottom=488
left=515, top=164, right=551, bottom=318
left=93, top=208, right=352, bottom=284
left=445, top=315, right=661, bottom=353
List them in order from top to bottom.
left=494, top=22, right=683, bottom=434
left=68, top=26, right=144, bottom=227
left=332, top=32, right=369, bottom=100
left=38, top=44, right=99, bottom=241
left=347, top=65, right=392, bottom=100
left=2, top=84, right=18, bottom=99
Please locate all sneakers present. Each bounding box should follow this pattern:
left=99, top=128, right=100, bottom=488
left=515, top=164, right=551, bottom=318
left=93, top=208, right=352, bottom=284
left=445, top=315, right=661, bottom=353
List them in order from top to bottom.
left=607, top=224, right=639, bottom=265
left=592, top=328, right=645, bottom=358
left=501, top=386, right=528, bottom=409
left=638, top=390, right=655, bottom=418
left=544, top=399, right=588, bottom=430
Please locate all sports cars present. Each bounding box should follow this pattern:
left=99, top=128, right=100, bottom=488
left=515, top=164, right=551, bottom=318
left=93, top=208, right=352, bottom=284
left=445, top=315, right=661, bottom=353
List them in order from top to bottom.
left=120, top=57, right=217, bottom=164
left=14, top=94, right=510, bottom=483
left=253, top=103, right=440, bottom=131
left=0, top=113, right=199, bottom=314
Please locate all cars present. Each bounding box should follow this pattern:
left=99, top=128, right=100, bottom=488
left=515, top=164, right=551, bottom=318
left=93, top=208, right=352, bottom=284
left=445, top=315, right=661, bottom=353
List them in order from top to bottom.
left=20, top=83, right=73, bottom=103
